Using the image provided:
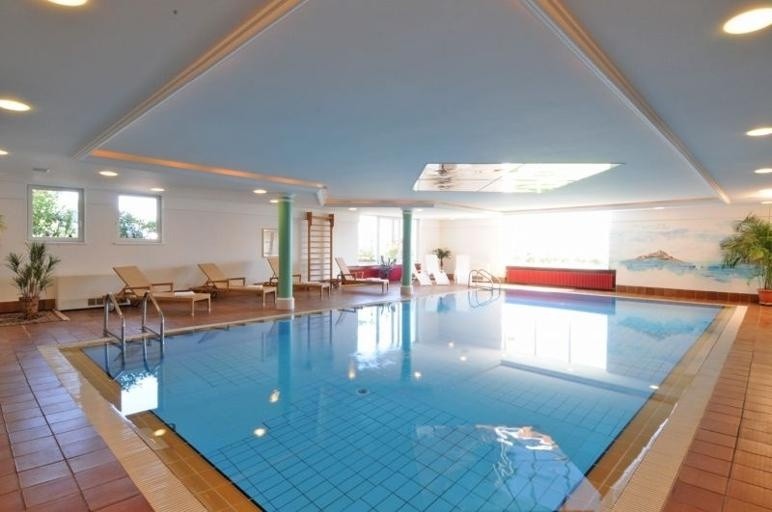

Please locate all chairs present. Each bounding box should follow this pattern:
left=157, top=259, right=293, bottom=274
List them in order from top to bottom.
left=263, top=255, right=331, bottom=299
left=423, top=255, right=450, bottom=286
left=334, top=256, right=390, bottom=294
left=410, top=260, right=432, bottom=286
left=190, top=262, right=277, bottom=309
left=113, top=266, right=211, bottom=317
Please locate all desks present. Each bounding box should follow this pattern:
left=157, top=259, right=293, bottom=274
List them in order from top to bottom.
left=505, top=266, right=616, bottom=291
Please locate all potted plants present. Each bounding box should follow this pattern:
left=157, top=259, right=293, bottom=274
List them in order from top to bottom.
left=720, top=211, right=772, bottom=306
left=3, top=241, right=62, bottom=320
